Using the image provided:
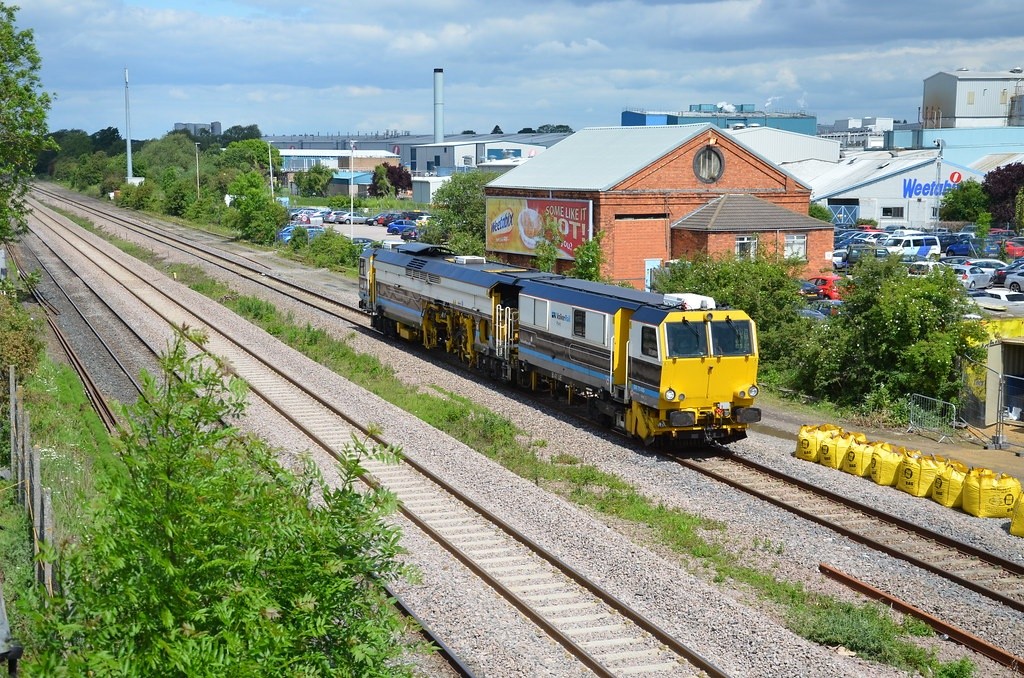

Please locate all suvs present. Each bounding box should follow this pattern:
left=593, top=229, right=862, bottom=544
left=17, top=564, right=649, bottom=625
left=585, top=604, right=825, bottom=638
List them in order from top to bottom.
left=353, top=238, right=382, bottom=249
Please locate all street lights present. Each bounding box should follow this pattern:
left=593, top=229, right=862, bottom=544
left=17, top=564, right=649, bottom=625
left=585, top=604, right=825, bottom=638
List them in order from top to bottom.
left=349, top=139, right=359, bottom=247
left=266, top=140, right=275, bottom=204
left=194, top=142, right=201, bottom=199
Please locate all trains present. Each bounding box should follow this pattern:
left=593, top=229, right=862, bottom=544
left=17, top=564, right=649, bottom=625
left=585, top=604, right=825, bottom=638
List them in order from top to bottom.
left=358, top=241, right=762, bottom=447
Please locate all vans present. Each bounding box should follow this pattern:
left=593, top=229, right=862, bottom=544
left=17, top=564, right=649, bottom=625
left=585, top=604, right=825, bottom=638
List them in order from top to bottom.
left=884, top=235, right=941, bottom=261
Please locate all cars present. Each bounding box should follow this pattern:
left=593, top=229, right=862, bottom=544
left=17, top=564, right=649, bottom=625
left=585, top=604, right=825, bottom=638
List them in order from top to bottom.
left=286, top=207, right=370, bottom=225
left=364, top=210, right=476, bottom=241
left=832, top=223, right=948, bottom=272
left=276, top=225, right=340, bottom=244
left=907, top=225, right=1024, bottom=312
left=790, top=276, right=856, bottom=320
